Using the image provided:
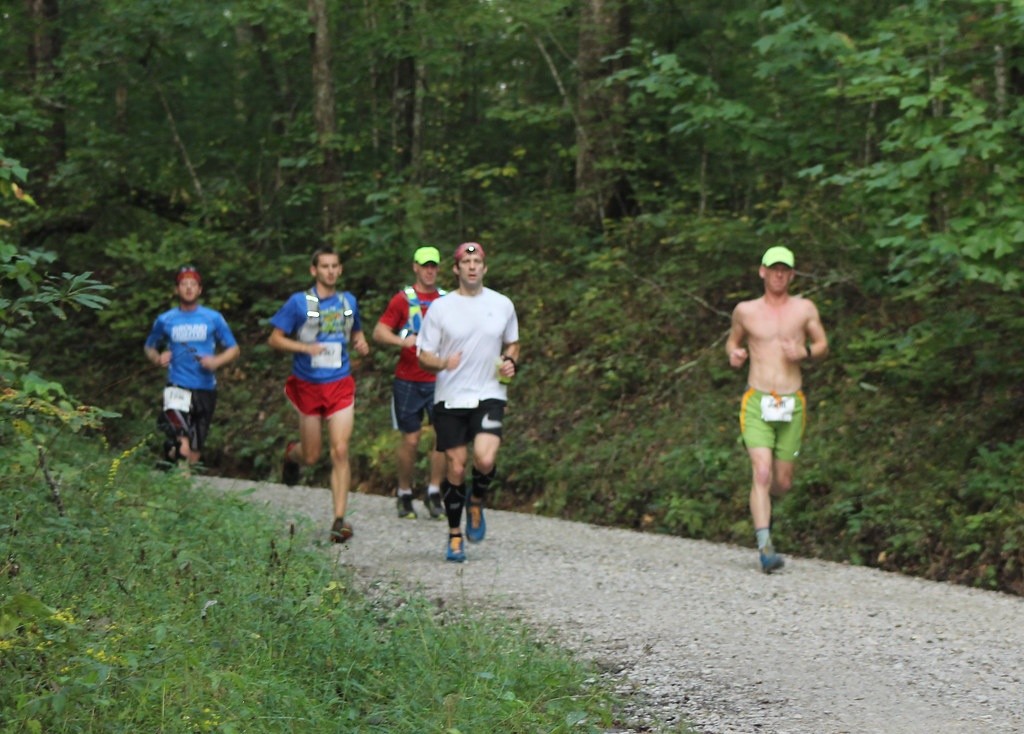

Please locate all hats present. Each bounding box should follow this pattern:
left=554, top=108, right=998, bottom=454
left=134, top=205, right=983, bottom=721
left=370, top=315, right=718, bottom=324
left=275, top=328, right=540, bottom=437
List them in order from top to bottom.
left=414, top=246, right=440, bottom=265
left=762, top=246, right=794, bottom=268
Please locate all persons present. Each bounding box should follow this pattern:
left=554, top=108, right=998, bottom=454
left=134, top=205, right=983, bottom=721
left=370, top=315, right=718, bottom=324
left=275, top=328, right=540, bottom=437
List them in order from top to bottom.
left=416, top=242, right=521, bottom=563
left=267, top=246, right=370, bottom=543
left=725, top=246, right=830, bottom=575
left=371, top=247, right=449, bottom=521
left=144, top=264, right=240, bottom=474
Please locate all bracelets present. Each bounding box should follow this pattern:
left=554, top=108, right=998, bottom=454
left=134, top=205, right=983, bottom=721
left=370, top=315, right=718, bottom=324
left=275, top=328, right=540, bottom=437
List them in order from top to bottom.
left=803, top=342, right=811, bottom=363
left=503, top=355, right=518, bottom=378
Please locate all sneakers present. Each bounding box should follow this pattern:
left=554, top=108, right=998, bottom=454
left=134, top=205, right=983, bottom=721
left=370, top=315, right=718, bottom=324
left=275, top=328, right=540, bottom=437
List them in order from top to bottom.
left=283, top=438, right=302, bottom=486
left=423, top=490, right=446, bottom=521
left=465, top=490, right=486, bottom=542
left=397, top=491, right=417, bottom=520
left=329, top=518, right=354, bottom=542
left=446, top=531, right=466, bottom=562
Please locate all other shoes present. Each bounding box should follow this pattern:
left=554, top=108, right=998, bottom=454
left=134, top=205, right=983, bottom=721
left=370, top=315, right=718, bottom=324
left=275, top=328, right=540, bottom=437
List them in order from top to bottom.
left=155, top=441, right=176, bottom=473
left=768, top=499, right=774, bottom=531
left=758, top=542, right=785, bottom=574
